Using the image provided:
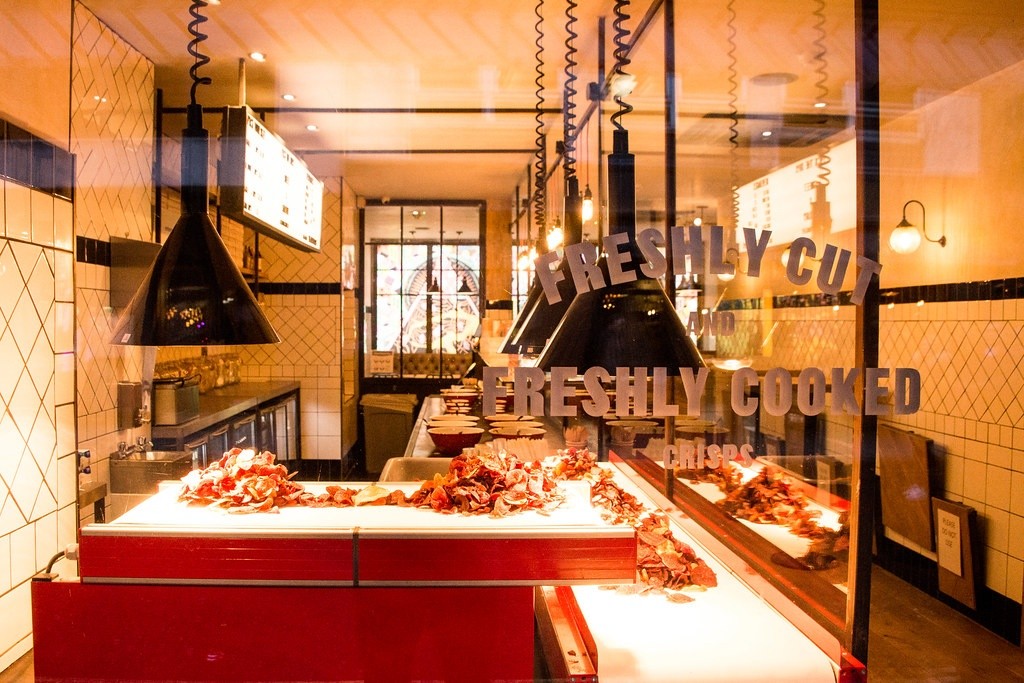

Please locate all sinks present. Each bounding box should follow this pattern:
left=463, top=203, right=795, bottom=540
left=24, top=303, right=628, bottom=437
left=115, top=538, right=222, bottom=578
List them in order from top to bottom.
left=109, top=450, right=194, bottom=494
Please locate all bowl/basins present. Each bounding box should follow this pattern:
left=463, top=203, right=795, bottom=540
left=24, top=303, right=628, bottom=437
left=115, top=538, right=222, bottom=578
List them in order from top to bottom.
left=440, top=389, right=476, bottom=393
left=496, top=390, right=514, bottom=405
left=440, top=393, right=478, bottom=410
left=445, top=399, right=472, bottom=416
left=496, top=399, right=507, bottom=412
left=429, top=416, right=479, bottom=420
left=484, top=416, right=535, bottom=421
left=425, top=421, right=477, bottom=430
left=489, top=421, right=543, bottom=427
left=427, top=427, right=485, bottom=454
left=488, top=427, right=547, bottom=440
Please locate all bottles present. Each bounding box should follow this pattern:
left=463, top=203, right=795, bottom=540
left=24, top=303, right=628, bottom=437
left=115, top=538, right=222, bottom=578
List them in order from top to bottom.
left=153, top=353, right=241, bottom=393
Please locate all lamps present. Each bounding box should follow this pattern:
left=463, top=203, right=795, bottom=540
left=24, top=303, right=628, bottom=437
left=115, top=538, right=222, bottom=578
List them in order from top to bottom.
left=109, top=0, right=281, bottom=346
left=499, top=1, right=710, bottom=376
left=890, top=199, right=946, bottom=254
left=782, top=246, right=825, bottom=268
left=716, top=248, right=747, bottom=283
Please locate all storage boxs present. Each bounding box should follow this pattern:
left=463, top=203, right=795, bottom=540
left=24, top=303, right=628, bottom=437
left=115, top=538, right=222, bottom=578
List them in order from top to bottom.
left=153, top=379, right=198, bottom=425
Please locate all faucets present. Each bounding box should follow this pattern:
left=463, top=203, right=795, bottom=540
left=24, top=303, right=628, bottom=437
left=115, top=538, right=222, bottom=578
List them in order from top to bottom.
left=134, top=405, right=151, bottom=425
left=134, top=436, right=154, bottom=453
left=116, top=441, right=144, bottom=459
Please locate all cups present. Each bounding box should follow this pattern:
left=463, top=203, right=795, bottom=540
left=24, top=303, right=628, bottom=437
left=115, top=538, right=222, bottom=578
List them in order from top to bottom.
left=566, top=440, right=588, bottom=449
left=611, top=441, right=634, bottom=460
